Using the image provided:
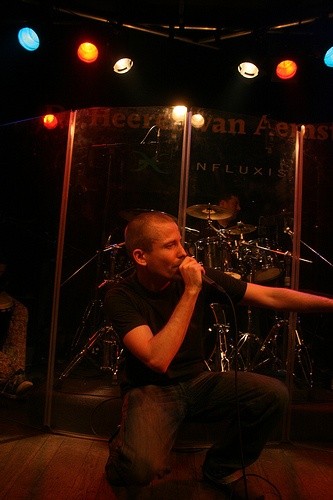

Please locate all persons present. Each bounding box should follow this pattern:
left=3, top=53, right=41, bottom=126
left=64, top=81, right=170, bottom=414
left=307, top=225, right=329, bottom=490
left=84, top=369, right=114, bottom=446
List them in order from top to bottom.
left=104, top=211, right=333, bottom=500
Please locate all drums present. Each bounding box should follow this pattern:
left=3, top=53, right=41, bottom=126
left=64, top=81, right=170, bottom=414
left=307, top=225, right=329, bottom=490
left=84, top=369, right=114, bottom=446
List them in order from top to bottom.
left=184, top=228, right=201, bottom=257
left=194, top=237, right=242, bottom=280
left=243, top=239, right=286, bottom=283
left=203, top=303, right=226, bottom=369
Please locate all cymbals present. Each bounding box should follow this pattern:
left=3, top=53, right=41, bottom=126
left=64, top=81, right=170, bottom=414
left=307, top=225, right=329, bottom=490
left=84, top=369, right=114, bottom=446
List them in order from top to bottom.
left=187, top=204, right=234, bottom=220
left=119, top=209, right=178, bottom=223
left=226, top=225, right=257, bottom=234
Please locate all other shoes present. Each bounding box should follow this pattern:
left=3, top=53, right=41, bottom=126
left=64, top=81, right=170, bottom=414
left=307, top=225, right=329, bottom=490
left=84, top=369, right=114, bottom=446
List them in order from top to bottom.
left=0, top=370, right=34, bottom=400
left=106, top=454, right=145, bottom=500
left=201, top=470, right=264, bottom=500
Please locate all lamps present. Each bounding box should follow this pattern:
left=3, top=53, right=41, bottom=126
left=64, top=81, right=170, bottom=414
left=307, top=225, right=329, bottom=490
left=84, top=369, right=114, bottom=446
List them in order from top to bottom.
left=17, top=2, right=53, bottom=53
left=238, top=16, right=333, bottom=83
left=75, top=19, right=110, bottom=63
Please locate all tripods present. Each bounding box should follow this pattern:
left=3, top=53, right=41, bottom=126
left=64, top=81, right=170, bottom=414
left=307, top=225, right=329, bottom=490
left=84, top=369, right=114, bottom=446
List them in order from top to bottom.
left=55, top=246, right=126, bottom=385
left=199, top=298, right=318, bottom=398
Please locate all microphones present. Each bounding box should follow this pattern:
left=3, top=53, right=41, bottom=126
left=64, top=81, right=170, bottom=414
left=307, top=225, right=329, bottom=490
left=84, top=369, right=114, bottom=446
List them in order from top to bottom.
left=178, top=262, right=218, bottom=287
